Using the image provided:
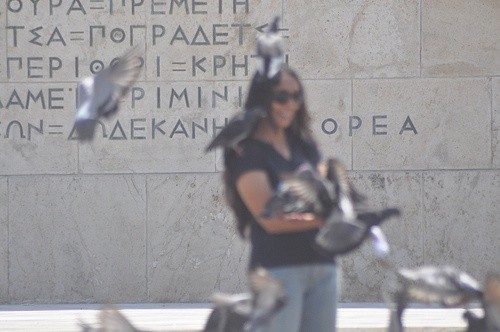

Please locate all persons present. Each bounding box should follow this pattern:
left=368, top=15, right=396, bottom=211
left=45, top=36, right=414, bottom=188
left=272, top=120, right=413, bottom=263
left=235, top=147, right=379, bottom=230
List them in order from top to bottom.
left=223, top=62, right=340, bottom=332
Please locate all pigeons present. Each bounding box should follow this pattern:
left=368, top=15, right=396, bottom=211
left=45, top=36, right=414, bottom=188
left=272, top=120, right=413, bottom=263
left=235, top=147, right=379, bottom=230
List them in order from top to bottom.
left=72, top=15, right=490, bottom=332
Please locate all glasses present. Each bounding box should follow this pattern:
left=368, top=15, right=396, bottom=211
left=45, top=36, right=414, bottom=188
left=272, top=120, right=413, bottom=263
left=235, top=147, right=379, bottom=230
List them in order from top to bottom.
left=272, top=90, right=304, bottom=104
